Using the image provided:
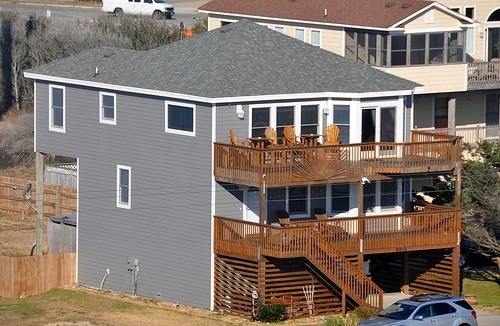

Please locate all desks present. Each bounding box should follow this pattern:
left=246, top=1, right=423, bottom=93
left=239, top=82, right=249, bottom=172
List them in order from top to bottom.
left=299, top=134, right=319, bottom=165
left=245, top=137, right=271, bottom=150
left=291, top=217, right=319, bottom=228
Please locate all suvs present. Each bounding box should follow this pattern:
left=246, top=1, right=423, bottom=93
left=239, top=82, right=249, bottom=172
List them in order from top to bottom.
left=357, top=293, right=479, bottom=326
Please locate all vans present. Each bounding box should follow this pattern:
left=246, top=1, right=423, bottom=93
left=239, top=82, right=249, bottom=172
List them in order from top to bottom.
left=102, top=0, right=173, bottom=20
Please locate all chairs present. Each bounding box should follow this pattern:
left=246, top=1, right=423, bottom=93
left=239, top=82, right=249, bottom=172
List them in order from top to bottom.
left=323, top=124, right=342, bottom=162
left=283, top=126, right=305, bottom=166
left=264, top=127, right=286, bottom=168
left=230, top=130, right=250, bottom=152
left=314, top=208, right=342, bottom=241
left=276, top=210, right=295, bottom=238
